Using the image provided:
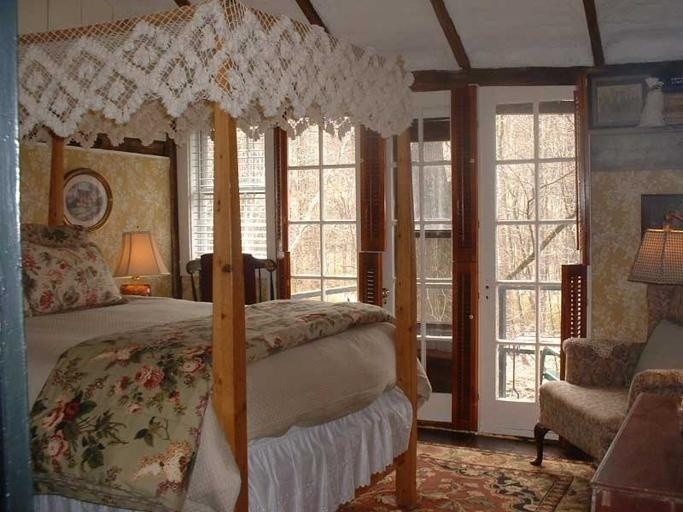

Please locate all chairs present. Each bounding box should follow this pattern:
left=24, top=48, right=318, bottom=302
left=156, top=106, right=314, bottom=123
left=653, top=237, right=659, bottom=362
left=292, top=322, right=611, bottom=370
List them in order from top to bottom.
left=185, top=253, right=278, bottom=306
left=529, top=282, right=682, bottom=467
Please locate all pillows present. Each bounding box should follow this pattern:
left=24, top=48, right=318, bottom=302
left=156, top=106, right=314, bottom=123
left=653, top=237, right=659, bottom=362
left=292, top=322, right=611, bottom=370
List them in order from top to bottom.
left=18, top=222, right=129, bottom=317
left=633, top=319, right=682, bottom=377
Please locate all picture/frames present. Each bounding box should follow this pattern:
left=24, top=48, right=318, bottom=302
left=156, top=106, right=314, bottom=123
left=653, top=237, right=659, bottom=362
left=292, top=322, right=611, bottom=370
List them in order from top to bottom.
left=587, top=71, right=652, bottom=128
left=61, top=168, right=114, bottom=232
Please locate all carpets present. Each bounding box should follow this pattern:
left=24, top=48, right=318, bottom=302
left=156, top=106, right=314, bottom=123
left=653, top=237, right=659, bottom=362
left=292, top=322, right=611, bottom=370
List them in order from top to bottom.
left=337, top=441, right=597, bottom=512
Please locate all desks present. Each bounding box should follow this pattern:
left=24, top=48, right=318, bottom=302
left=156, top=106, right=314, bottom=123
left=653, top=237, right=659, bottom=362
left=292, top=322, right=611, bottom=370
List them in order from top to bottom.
left=588, top=392, right=683, bottom=512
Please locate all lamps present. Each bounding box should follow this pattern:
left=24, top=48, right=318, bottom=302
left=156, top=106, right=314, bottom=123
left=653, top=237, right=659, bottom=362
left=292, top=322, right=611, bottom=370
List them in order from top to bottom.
left=626, top=207, right=683, bottom=285
left=112, top=225, right=172, bottom=298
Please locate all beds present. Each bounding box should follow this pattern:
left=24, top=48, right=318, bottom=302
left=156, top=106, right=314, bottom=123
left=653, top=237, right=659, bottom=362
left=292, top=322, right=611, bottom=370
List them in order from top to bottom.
left=15, top=0, right=433, bottom=512
